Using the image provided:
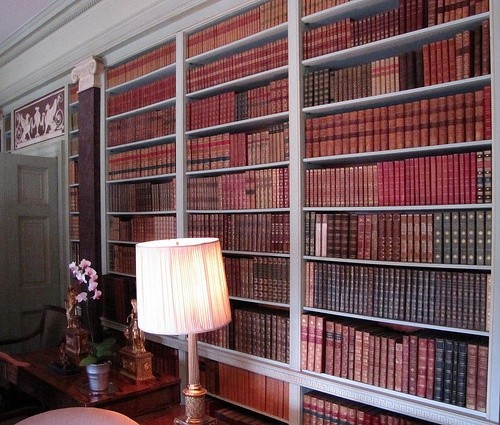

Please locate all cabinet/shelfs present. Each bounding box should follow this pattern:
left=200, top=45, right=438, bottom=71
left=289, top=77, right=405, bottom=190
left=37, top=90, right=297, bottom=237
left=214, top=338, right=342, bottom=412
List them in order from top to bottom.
left=68, top=0, right=500, bottom=425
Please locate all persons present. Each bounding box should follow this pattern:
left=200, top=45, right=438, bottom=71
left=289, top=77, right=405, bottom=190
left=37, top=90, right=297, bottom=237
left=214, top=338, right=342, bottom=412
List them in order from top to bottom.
left=65, top=286, right=81, bottom=328
left=125, top=300, right=147, bottom=355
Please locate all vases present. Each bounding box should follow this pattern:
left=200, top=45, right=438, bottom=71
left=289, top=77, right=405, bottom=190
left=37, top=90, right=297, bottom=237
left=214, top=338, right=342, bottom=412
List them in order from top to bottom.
left=85, top=363, right=109, bottom=392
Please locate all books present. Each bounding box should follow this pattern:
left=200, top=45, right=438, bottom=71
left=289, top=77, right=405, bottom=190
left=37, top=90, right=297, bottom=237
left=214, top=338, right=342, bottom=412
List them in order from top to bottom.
left=299, top=0, right=492, bottom=425
left=185, top=1, right=290, bottom=425
left=103, top=34, right=176, bottom=405
left=68, top=82, right=81, bottom=295
left=4, top=114, right=12, bottom=153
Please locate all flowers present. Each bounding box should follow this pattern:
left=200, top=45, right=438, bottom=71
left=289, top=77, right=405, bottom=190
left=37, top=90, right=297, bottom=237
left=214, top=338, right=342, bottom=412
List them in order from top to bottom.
left=69, top=258, right=118, bottom=366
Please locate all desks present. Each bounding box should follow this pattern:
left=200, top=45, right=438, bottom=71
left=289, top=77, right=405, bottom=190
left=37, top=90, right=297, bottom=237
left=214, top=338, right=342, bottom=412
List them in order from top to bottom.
left=3, top=351, right=180, bottom=418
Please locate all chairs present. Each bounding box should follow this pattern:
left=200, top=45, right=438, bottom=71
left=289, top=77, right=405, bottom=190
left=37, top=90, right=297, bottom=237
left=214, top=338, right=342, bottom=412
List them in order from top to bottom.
left=0, top=304, right=66, bottom=348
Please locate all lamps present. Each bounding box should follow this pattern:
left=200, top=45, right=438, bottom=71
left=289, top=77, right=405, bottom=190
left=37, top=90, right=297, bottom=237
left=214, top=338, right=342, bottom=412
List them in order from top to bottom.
left=135, top=237, right=232, bottom=425
